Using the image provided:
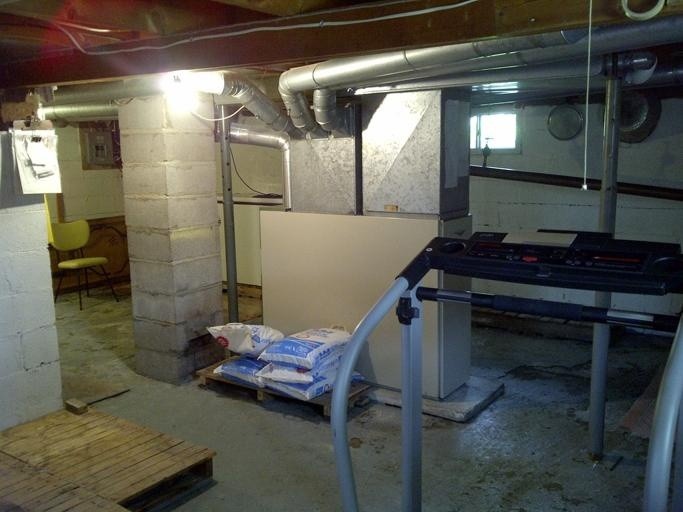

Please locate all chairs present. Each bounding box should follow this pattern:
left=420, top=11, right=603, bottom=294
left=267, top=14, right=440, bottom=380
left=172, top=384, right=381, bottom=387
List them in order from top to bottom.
left=48, top=219, right=120, bottom=310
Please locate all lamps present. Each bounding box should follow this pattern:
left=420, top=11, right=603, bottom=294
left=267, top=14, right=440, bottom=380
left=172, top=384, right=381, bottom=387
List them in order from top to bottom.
left=157, top=68, right=206, bottom=119
